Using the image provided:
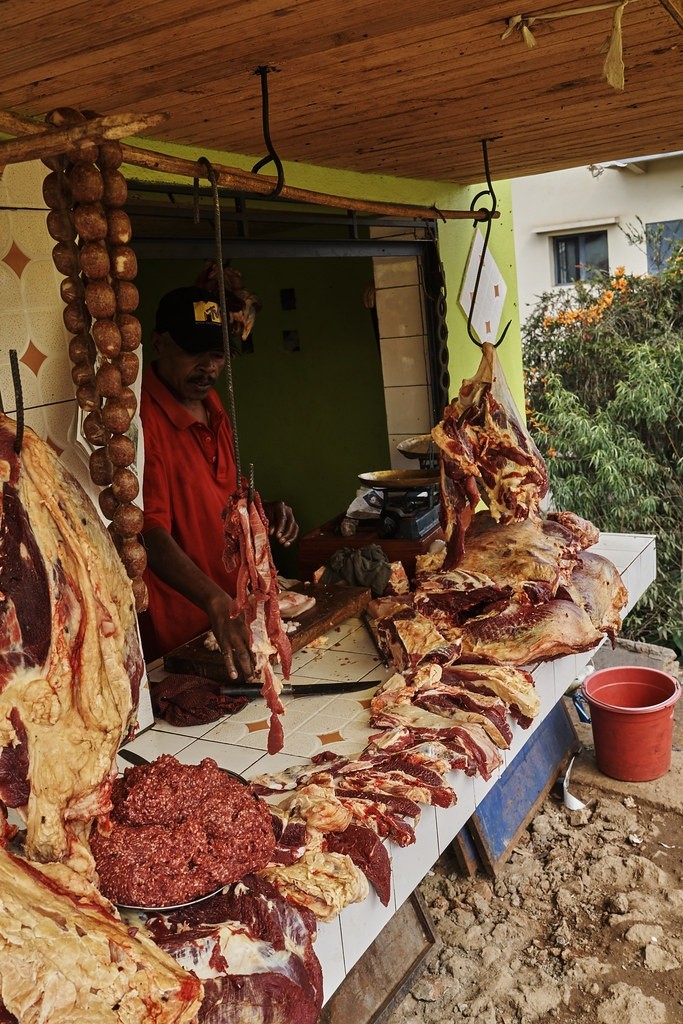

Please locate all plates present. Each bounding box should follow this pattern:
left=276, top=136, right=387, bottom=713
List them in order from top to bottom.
left=397, top=434, right=441, bottom=459
left=357, top=470, right=441, bottom=488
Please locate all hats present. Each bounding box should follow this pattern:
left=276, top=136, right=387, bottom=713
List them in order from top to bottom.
left=155, top=287, right=243, bottom=357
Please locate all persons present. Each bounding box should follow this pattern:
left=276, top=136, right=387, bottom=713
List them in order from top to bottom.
left=140, top=286, right=300, bottom=681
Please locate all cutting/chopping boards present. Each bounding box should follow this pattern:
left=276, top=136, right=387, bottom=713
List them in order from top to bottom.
left=161, top=583, right=372, bottom=681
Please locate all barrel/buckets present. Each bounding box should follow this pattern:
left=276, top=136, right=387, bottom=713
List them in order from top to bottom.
left=581, top=666, right=681, bottom=782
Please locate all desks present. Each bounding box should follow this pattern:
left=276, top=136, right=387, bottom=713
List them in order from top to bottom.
left=114, top=533, right=657, bottom=1010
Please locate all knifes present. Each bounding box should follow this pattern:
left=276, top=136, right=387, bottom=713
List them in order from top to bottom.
left=218, top=680, right=382, bottom=696
